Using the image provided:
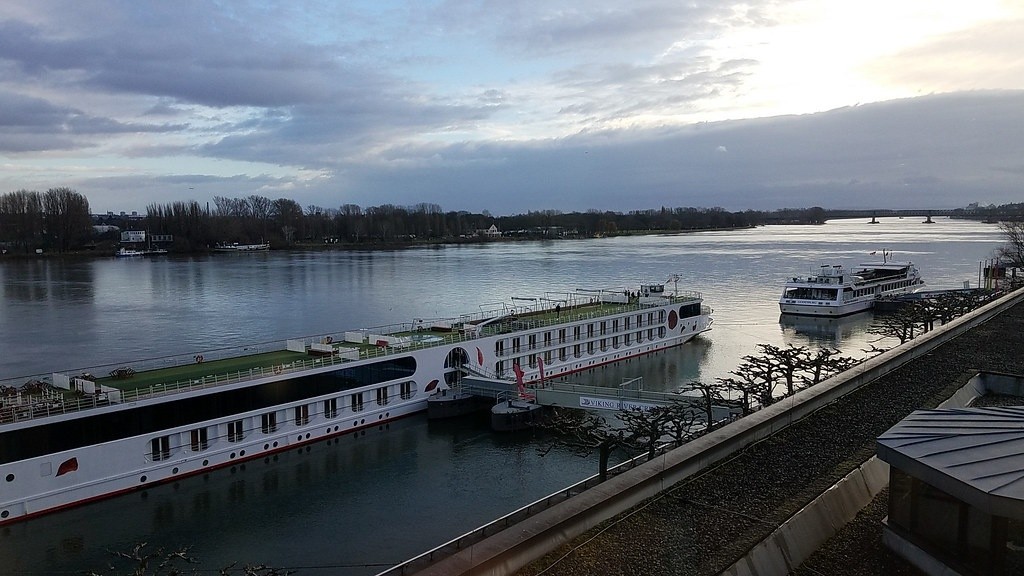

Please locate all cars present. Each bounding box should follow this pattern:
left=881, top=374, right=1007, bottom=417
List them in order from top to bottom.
left=1004, top=267, right=1024, bottom=278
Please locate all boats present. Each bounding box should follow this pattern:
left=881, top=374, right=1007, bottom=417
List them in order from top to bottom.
left=208, top=238, right=270, bottom=251
left=116, top=248, right=168, bottom=257
left=779, top=249, right=927, bottom=316
left=0, top=276, right=716, bottom=526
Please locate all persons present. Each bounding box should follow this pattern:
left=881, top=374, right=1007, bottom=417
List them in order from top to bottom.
left=625, top=289, right=649, bottom=302
left=556, top=304, right=561, bottom=318
